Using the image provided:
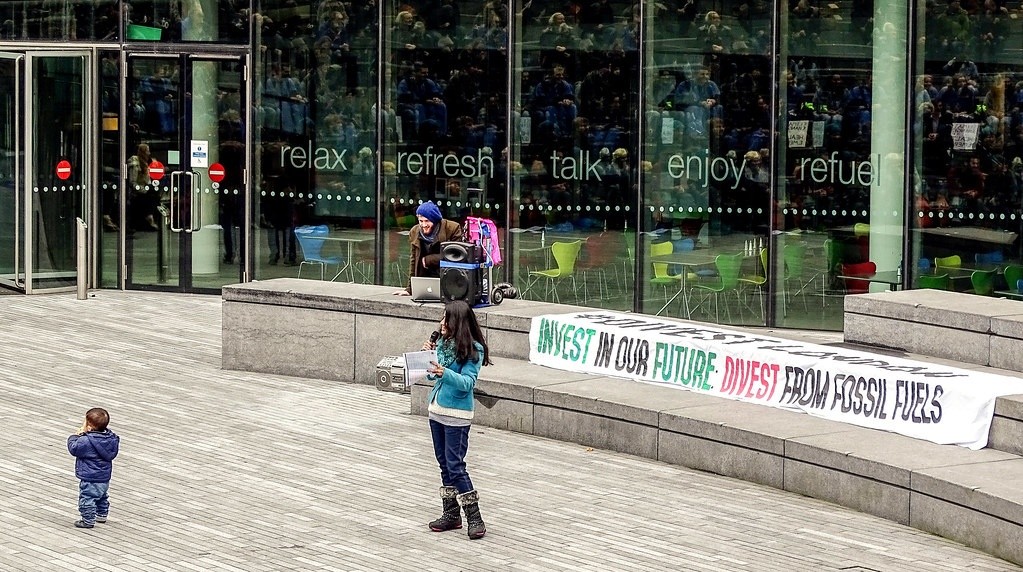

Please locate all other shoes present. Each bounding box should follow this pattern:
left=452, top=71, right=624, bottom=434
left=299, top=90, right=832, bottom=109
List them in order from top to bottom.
left=75, top=520, right=94, bottom=529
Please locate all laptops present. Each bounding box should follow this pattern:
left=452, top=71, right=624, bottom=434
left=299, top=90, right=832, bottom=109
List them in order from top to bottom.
left=411, top=277, right=440, bottom=302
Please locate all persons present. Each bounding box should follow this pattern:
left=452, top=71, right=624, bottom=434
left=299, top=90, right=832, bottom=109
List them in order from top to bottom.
left=0, top=0, right=1023, bottom=266
left=392, top=201, right=460, bottom=296
left=420, top=300, right=495, bottom=540
left=67, top=407, right=120, bottom=528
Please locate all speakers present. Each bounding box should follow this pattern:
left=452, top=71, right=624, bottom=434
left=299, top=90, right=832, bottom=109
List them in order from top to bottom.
left=439, top=242, right=482, bottom=307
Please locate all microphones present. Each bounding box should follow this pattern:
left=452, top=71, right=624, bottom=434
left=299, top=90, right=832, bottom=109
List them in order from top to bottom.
left=429, top=331, right=440, bottom=344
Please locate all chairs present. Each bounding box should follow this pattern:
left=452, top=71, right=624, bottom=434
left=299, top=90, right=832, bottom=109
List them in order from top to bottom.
left=295, top=225, right=340, bottom=278
left=524, top=225, right=1023, bottom=324
left=363, top=229, right=400, bottom=286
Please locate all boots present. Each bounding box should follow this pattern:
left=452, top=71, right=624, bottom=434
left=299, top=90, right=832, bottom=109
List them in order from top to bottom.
left=145, top=215, right=159, bottom=232
left=429, top=486, right=462, bottom=531
left=456, top=489, right=486, bottom=539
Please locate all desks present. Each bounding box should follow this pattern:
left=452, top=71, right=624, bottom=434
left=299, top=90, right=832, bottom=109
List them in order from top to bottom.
left=315, top=229, right=374, bottom=282
left=544, top=232, right=588, bottom=302
left=520, top=242, right=551, bottom=300
left=652, top=245, right=718, bottom=317
left=685, top=247, right=760, bottom=259
left=836, top=263, right=1003, bottom=290
left=994, top=291, right=1023, bottom=296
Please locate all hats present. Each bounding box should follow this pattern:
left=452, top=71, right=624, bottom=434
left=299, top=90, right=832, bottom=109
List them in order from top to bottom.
left=417, top=201, right=442, bottom=224
